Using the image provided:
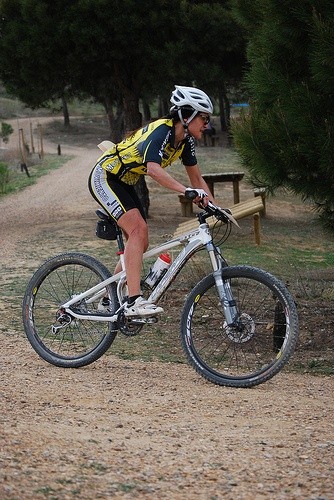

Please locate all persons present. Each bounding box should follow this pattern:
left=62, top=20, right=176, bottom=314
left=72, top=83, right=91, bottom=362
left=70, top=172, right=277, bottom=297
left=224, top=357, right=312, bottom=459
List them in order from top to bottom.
left=87, top=85, right=232, bottom=320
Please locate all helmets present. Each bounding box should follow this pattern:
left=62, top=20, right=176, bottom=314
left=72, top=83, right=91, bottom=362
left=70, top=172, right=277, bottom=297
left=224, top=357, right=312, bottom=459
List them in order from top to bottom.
left=170, top=85, right=214, bottom=114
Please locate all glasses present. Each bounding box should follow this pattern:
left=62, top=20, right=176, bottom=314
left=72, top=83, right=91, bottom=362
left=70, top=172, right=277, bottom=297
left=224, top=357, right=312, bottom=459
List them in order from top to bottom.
left=198, top=114, right=211, bottom=124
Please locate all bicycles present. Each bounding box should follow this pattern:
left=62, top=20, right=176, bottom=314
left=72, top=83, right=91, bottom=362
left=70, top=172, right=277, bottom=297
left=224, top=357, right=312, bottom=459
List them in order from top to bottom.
left=21, top=188, right=300, bottom=388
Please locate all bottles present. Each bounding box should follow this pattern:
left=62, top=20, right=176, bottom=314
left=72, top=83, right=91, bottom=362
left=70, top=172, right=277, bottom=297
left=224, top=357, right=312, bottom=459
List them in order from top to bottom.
left=145, top=252, right=170, bottom=286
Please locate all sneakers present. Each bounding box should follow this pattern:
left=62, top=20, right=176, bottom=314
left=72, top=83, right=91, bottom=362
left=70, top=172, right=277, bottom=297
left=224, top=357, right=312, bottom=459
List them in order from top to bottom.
left=124, top=296, right=164, bottom=317
left=97, top=297, right=109, bottom=309
